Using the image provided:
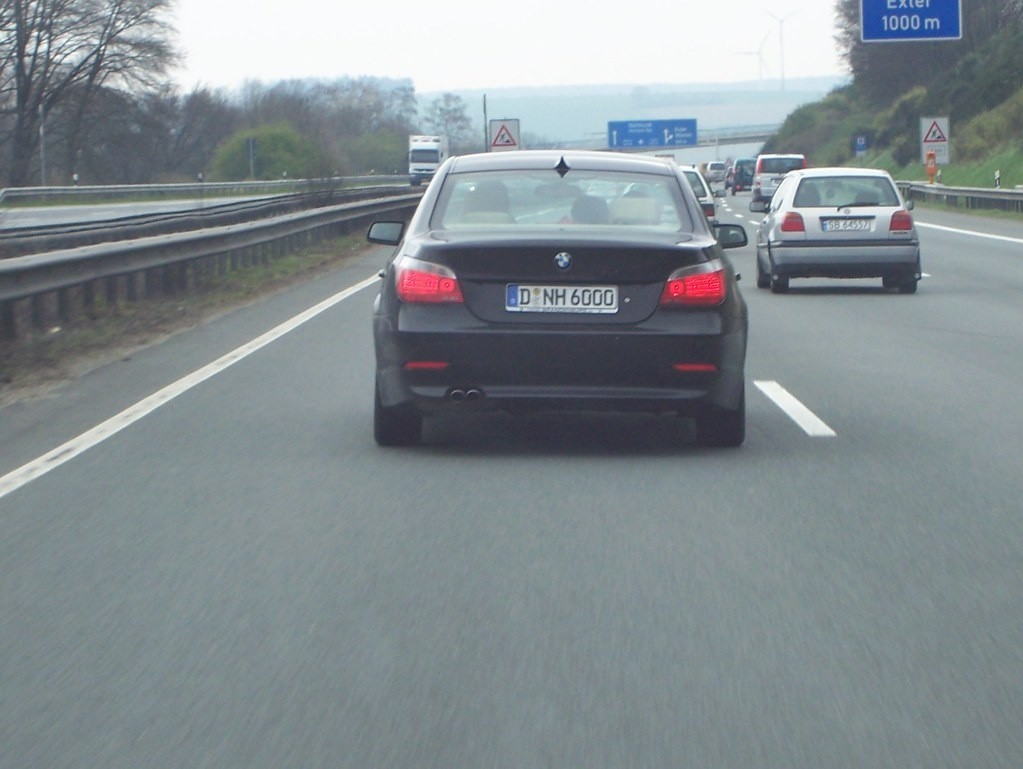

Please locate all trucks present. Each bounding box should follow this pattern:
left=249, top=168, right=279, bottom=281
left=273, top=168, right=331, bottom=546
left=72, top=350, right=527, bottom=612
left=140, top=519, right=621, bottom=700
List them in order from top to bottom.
left=407, top=134, right=449, bottom=184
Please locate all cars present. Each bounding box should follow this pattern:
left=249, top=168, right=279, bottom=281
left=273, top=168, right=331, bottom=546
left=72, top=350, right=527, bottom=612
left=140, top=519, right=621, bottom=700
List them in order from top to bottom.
left=362, top=148, right=748, bottom=449
left=675, top=158, right=759, bottom=229
left=749, top=167, right=922, bottom=294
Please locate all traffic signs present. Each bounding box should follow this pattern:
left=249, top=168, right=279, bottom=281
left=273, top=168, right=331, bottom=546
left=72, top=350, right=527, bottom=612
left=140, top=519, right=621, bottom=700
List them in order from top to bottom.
left=860, top=0, right=964, bottom=44
left=606, top=118, right=698, bottom=150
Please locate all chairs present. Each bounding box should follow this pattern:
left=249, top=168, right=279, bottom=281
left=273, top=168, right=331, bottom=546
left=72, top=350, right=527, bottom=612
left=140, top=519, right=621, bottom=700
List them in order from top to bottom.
left=610, top=197, right=662, bottom=226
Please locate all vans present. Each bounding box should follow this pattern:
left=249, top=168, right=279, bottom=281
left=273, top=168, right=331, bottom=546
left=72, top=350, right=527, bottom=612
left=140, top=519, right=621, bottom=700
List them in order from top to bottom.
left=748, top=153, right=809, bottom=212
